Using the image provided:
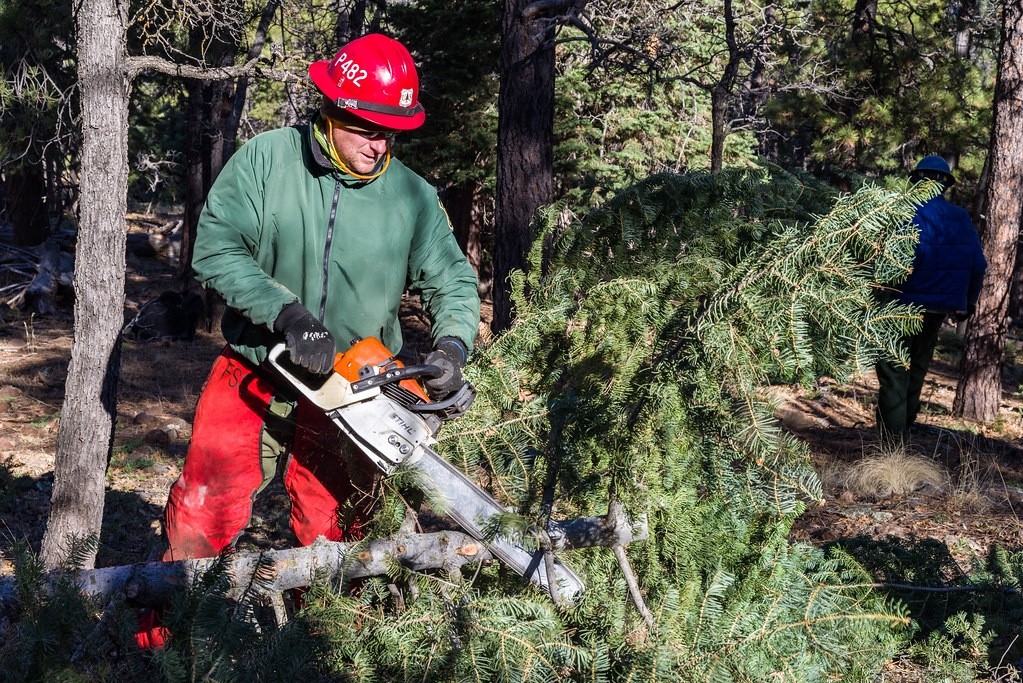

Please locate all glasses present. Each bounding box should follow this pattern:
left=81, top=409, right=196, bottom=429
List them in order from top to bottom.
left=350, top=129, right=397, bottom=139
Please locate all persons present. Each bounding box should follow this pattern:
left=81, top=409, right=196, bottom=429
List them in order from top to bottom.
left=135, top=29, right=483, bottom=652
left=875, top=156, right=989, bottom=451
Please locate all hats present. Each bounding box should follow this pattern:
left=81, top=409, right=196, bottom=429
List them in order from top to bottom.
left=908, top=155, right=956, bottom=190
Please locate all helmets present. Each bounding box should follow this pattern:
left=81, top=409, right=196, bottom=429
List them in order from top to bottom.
left=308, top=33, right=425, bottom=133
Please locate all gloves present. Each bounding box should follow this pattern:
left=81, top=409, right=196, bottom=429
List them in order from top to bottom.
left=273, top=301, right=336, bottom=375
left=421, top=336, right=467, bottom=401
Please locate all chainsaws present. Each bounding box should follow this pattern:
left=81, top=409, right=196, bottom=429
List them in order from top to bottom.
left=267, top=333, right=586, bottom=615
